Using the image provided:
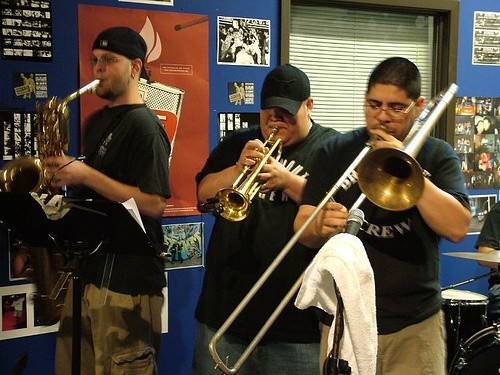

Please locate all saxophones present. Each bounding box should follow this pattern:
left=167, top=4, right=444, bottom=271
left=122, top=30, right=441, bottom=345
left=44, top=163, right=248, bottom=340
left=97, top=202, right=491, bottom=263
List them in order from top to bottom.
left=0, top=79, right=101, bottom=196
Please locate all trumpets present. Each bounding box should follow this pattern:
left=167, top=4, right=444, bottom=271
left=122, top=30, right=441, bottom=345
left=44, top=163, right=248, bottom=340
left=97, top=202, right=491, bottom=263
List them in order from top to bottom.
left=212, top=125, right=284, bottom=222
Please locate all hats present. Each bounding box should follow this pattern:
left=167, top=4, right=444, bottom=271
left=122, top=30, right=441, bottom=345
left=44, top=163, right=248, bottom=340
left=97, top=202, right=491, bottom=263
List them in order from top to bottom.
left=92, top=26, right=150, bottom=81
left=260, top=64, right=310, bottom=115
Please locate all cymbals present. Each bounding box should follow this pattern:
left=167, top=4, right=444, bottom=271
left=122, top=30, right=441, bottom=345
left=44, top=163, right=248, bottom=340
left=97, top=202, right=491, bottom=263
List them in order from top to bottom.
left=441, top=250, right=500, bottom=263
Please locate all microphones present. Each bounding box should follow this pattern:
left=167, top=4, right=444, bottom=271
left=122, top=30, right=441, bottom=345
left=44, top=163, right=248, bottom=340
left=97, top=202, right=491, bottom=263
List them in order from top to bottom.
left=346, top=208, right=365, bottom=235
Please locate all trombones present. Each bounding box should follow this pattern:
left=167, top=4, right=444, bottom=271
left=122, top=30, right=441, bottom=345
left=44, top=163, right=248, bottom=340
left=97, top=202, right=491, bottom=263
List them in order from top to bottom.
left=205, top=82, right=460, bottom=374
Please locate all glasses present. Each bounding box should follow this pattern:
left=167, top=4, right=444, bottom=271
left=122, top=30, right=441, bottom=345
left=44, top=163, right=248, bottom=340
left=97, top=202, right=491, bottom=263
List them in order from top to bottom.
left=88, top=56, right=137, bottom=66
left=363, top=97, right=416, bottom=120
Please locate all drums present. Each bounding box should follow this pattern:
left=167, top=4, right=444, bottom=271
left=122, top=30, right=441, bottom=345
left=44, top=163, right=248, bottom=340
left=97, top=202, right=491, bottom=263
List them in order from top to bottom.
left=439, top=287, right=489, bottom=375
left=446, top=322, right=500, bottom=375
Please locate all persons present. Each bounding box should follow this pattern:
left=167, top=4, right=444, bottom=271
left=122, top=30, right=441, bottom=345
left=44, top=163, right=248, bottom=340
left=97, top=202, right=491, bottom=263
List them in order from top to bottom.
left=42, top=26, right=170, bottom=375
left=293, top=58, right=473, bottom=375
left=191, top=63, right=342, bottom=375
left=455, top=97, right=500, bottom=190
left=473, top=201, right=500, bottom=328
left=10, top=294, right=26, bottom=318
